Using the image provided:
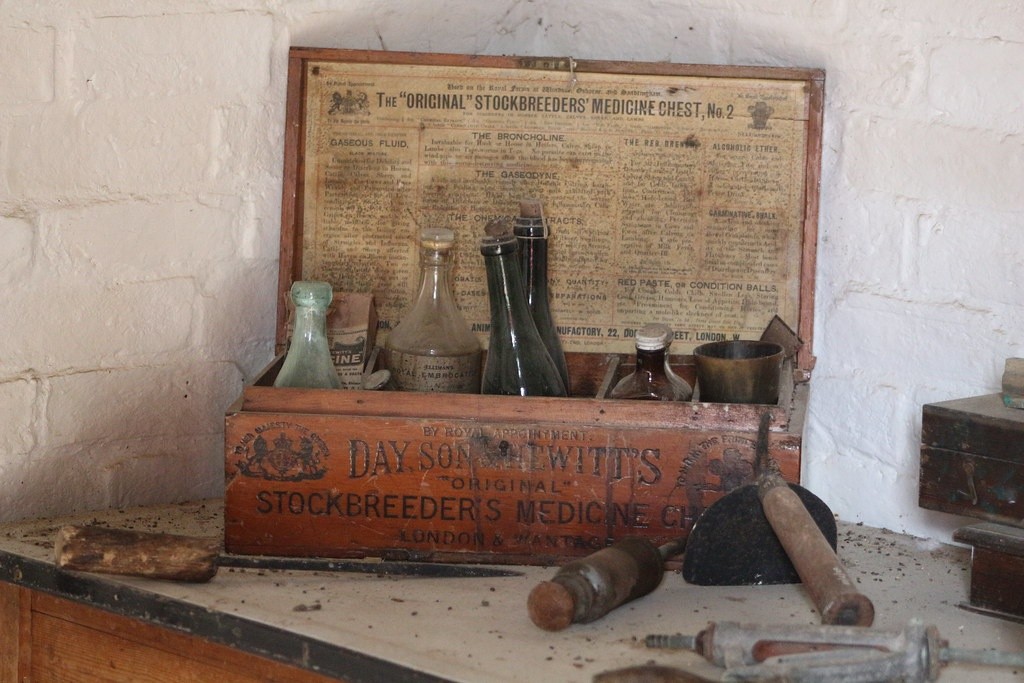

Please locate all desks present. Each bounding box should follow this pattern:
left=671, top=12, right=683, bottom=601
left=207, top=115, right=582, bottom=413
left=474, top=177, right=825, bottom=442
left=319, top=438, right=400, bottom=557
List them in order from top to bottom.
left=1, top=493, right=1024, bottom=682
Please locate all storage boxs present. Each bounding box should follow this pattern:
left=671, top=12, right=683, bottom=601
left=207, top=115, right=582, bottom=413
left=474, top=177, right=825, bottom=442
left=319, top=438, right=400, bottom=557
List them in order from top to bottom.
left=219, top=45, right=827, bottom=581
left=918, top=392, right=1024, bottom=529
left=952, top=523, right=1024, bottom=624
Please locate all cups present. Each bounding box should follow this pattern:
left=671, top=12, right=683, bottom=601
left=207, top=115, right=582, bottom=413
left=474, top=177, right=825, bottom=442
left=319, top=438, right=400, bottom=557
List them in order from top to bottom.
left=693, top=341, right=784, bottom=405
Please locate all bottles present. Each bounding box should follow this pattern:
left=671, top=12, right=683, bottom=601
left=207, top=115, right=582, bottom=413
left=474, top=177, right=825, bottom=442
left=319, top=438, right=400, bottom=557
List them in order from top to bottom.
left=512, top=202, right=571, bottom=398
left=480, top=219, right=567, bottom=398
left=384, top=228, right=482, bottom=392
left=272, top=280, right=343, bottom=387
left=611, top=325, right=693, bottom=401
left=528, top=535, right=664, bottom=632
